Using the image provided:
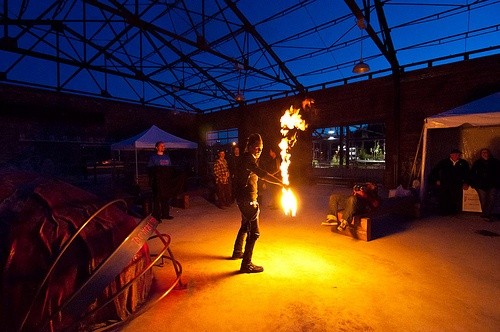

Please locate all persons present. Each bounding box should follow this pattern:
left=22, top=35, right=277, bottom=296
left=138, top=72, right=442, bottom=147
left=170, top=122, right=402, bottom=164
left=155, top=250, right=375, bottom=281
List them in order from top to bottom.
left=232, top=133, right=269, bottom=272
left=147, top=141, right=180, bottom=222
left=211, top=145, right=282, bottom=210
left=429, top=148, right=500, bottom=222
left=321, top=180, right=385, bottom=232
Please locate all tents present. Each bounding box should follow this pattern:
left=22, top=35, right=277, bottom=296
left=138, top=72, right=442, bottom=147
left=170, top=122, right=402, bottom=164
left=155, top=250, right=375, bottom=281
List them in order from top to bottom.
left=407, top=92, right=500, bottom=205
left=110, top=123, right=199, bottom=185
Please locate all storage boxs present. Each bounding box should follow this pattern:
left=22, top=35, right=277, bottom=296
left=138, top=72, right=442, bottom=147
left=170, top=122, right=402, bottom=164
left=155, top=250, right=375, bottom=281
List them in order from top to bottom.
left=462, top=185, right=483, bottom=212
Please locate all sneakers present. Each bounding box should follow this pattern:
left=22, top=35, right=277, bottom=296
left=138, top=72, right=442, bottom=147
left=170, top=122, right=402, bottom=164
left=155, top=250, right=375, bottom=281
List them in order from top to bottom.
left=322, top=218, right=338, bottom=225
left=337, top=221, right=346, bottom=231
left=231, top=252, right=244, bottom=260
left=240, top=264, right=263, bottom=272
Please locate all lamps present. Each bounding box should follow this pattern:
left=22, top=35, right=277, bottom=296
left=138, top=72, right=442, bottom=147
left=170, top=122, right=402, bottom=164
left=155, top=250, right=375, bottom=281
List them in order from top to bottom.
left=352, top=17, right=370, bottom=74
left=235, top=64, right=245, bottom=101
